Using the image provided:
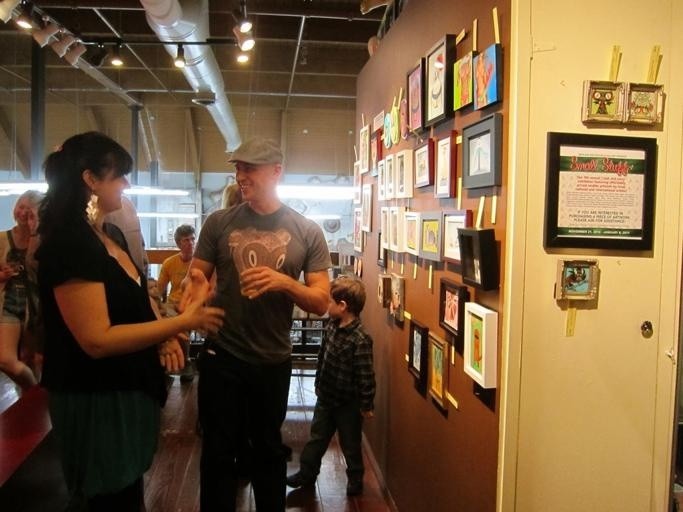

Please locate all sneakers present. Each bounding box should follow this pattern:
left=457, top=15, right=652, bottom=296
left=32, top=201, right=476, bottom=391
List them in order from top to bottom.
left=179, top=363, right=195, bottom=382
left=286, top=470, right=316, bottom=487
left=346, top=476, right=363, bottom=495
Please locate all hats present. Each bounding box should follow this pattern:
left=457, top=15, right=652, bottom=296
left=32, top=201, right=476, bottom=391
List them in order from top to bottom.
left=227, top=136, right=283, bottom=166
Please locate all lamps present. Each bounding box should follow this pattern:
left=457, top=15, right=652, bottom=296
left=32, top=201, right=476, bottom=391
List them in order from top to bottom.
left=0, top=0, right=89, bottom=67
left=229, top=2, right=255, bottom=67
left=110, top=41, right=187, bottom=68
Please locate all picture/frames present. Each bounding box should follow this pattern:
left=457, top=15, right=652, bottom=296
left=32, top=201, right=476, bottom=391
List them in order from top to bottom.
left=581, top=78, right=626, bottom=127
left=623, top=80, right=666, bottom=128
left=542, top=133, right=658, bottom=249
left=553, top=256, right=601, bottom=303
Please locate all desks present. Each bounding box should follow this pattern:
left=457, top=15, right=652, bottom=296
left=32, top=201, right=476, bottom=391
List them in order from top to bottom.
left=0, top=372, right=55, bottom=490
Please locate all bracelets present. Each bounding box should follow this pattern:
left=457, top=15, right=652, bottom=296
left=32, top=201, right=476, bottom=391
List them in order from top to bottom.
left=29, top=233, right=38, bottom=237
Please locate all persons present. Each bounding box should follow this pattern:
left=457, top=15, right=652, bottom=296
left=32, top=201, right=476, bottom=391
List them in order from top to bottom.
left=33, top=131, right=225, bottom=511
left=177, top=137, right=329, bottom=511
left=219, top=182, right=249, bottom=207
left=0, top=189, right=43, bottom=395
left=102, top=195, right=149, bottom=276
left=158, top=225, right=217, bottom=384
left=284, top=274, right=376, bottom=496
left=145, top=278, right=167, bottom=315
